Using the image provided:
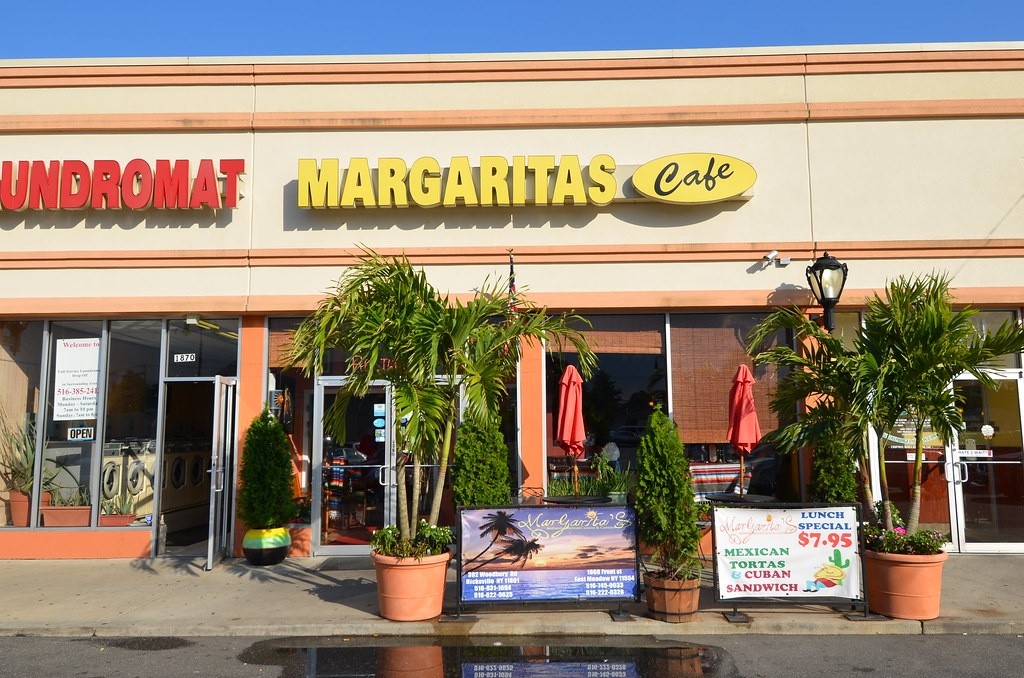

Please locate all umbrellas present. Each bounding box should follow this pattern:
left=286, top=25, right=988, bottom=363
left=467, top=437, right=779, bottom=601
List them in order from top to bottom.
left=556, top=365, right=587, bottom=499
left=726, top=365, right=762, bottom=498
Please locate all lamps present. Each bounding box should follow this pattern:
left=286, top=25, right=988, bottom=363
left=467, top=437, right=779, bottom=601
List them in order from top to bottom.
left=804, top=251, right=850, bottom=336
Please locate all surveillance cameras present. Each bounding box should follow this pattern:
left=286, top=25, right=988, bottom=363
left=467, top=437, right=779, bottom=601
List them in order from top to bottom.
left=763, top=250, right=779, bottom=261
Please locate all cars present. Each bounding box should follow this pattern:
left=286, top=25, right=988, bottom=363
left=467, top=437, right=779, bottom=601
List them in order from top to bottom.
left=608, top=425, right=648, bottom=445
left=326, top=447, right=368, bottom=463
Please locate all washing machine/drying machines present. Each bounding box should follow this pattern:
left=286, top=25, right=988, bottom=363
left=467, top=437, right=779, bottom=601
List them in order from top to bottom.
left=121, top=433, right=210, bottom=519
left=99, top=443, right=125, bottom=515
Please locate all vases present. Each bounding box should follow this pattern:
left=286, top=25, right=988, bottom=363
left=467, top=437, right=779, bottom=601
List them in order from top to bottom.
left=374, top=645, right=444, bottom=678
left=638, top=648, right=704, bottom=678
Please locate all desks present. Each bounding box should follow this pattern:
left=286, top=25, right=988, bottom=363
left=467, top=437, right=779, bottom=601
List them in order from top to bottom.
left=704, top=493, right=776, bottom=504
left=542, top=494, right=613, bottom=505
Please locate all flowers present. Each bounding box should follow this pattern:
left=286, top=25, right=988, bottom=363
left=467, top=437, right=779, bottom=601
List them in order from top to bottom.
left=368, top=519, right=457, bottom=563
left=857, top=521, right=949, bottom=556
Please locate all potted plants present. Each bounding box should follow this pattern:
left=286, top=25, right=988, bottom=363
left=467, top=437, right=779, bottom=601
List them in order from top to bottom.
left=276, top=239, right=603, bottom=622
left=746, top=267, right=1024, bottom=623
left=0, top=396, right=69, bottom=528
left=234, top=399, right=296, bottom=566
left=631, top=404, right=712, bottom=624
left=604, top=459, right=636, bottom=503
left=37, top=482, right=92, bottom=527
left=99, top=488, right=138, bottom=527
